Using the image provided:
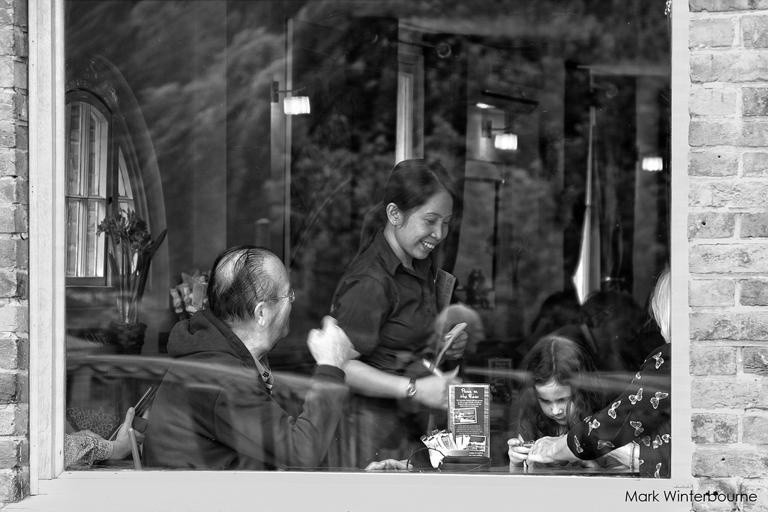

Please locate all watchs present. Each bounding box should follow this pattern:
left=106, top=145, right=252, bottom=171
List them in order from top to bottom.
left=407, top=375, right=418, bottom=401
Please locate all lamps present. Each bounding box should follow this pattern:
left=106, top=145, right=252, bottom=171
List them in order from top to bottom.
left=267, top=79, right=311, bottom=117
left=478, top=118, right=519, bottom=152
left=620, top=148, right=664, bottom=174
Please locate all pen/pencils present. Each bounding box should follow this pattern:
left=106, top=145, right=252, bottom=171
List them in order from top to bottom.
left=421, top=358, right=448, bottom=379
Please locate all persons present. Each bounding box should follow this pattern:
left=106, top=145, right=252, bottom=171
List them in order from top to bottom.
left=325, top=158, right=468, bottom=473
left=143, top=244, right=413, bottom=470
left=525, top=269, right=671, bottom=473
left=506, top=291, right=645, bottom=408
left=406, top=300, right=486, bottom=382
left=508, top=336, right=607, bottom=469
left=64, top=399, right=149, bottom=470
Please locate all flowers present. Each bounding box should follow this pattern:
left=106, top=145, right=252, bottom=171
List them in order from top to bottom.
left=92, top=203, right=169, bottom=323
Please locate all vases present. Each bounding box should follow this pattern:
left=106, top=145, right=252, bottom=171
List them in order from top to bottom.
left=101, top=316, right=150, bottom=357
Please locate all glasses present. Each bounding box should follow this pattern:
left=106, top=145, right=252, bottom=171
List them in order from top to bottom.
left=260, top=287, right=297, bottom=305
